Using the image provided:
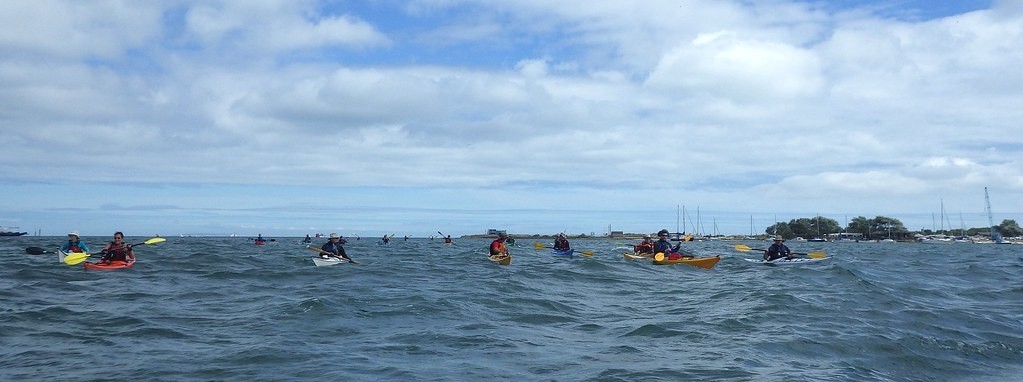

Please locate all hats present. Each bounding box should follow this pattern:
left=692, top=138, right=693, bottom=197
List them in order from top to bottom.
left=657, top=229, right=669, bottom=238
left=68, top=232, right=80, bottom=239
left=560, top=232, right=566, bottom=239
left=328, top=233, right=339, bottom=239
left=643, top=234, right=653, bottom=238
left=773, top=235, right=784, bottom=241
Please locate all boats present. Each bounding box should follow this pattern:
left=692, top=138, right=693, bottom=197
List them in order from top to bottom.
left=0, top=225, right=27, bottom=237
left=444, top=241, right=451, bottom=247
left=507, top=242, right=515, bottom=247
left=612, top=246, right=694, bottom=259
left=56, top=247, right=89, bottom=264
left=84, top=248, right=137, bottom=271
left=550, top=248, right=575, bottom=256
left=488, top=251, right=511, bottom=266
left=623, top=252, right=721, bottom=270
left=311, top=254, right=345, bottom=268
left=744, top=255, right=833, bottom=268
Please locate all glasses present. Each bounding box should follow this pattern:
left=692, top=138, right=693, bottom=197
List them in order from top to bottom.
left=661, top=234, right=668, bottom=237
left=114, top=237, right=121, bottom=240
left=334, top=238, right=339, bottom=240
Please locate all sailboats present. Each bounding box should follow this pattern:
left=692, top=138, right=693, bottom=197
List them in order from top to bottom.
left=911, top=197, right=1011, bottom=245
left=668, top=203, right=894, bottom=242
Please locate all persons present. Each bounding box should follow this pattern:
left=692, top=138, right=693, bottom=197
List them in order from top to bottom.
left=654, top=229, right=681, bottom=257
left=60, top=231, right=89, bottom=254
left=101, top=231, right=135, bottom=263
left=490, top=232, right=507, bottom=255
left=554, top=232, right=570, bottom=251
left=319, top=233, right=354, bottom=263
left=258, top=234, right=263, bottom=241
left=305, top=234, right=311, bottom=243
left=634, top=234, right=654, bottom=255
left=382, top=235, right=389, bottom=244
left=443, top=235, right=451, bottom=243
left=506, top=235, right=515, bottom=243
left=768, top=235, right=790, bottom=260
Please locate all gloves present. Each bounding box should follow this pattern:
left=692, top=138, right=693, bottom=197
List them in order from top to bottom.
left=328, top=252, right=335, bottom=257
left=347, top=258, right=353, bottom=263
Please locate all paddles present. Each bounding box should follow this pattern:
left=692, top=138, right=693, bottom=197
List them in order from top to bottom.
left=438, top=231, right=457, bottom=244
left=376, top=233, right=394, bottom=244
left=654, top=233, right=692, bottom=262
left=407, top=235, right=412, bottom=238
left=63, top=237, right=167, bottom=265
left=250, top=237, right=275, bottom=242
left=614, top=241, right=646, bottom=247
left=534, top=242, right=593, bottom=257
left=735, top=244, right=826, bottom=259
left=306, top=245, right=359, bottom=263
left=25, top=246, right=58, bottom=256
